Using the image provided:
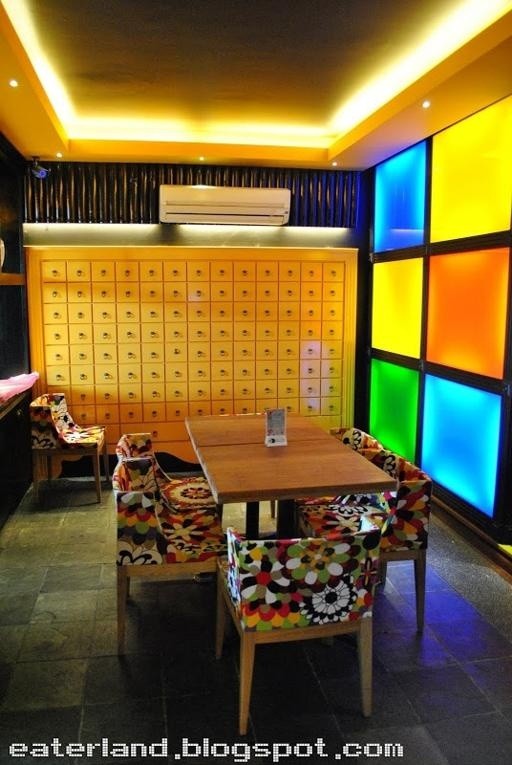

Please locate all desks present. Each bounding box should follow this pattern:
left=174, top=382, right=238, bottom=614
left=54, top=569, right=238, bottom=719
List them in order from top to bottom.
left=184, top=412, right=397, bottom=541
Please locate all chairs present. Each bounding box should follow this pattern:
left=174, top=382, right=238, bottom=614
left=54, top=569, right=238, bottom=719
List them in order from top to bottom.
left=29, top=392, right=110, bottom=503
left=271, top=426, right=384, bottom=518
left=115, top=432, right=222, bottom=518
left=295, top=449, right=434, bottom=634
left=112, top=458, right=228, bottom=656
left=215, top=513, right=382, bottom=736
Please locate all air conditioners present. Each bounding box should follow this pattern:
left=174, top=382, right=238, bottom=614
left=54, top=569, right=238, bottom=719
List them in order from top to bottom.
left=159, top=184, right=292, bottom=226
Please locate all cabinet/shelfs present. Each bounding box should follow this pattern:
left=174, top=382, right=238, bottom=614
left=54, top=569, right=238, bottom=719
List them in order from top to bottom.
left=0, top=130, right=33, bottom=531
left=23, top=244, right=359, bottom=482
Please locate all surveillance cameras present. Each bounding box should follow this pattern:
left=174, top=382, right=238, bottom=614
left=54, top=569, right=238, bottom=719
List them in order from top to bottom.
left=30, top=160, right=49, bottom=178
